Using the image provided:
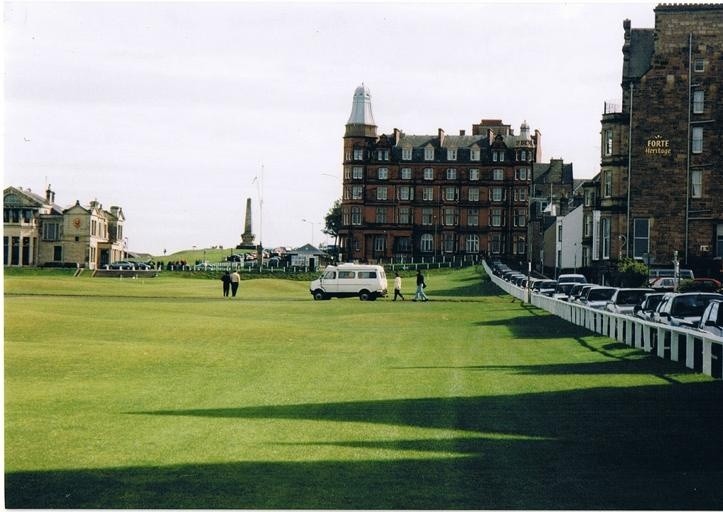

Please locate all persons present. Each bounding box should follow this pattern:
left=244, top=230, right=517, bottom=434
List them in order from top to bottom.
left=228, top=269, right=240, bottom=297
left=410, top=269, right=430, bottom=301
left=219, top=271, right=231, bottom=297
left=390, top=272, right=404, bottom=301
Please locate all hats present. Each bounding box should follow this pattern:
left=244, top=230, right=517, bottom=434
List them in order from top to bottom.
left=227, top=251, right=270, bottom=261
left=103, top=261, right=151, bottom=269
left=492, top=261, right=723, bottom=367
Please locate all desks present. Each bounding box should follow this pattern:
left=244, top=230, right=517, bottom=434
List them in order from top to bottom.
left=617, top=235, right=626, bottom=259
left=125, top=237, right=129, bottom=261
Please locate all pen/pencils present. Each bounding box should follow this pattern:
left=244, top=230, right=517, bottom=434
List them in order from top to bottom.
left=310, top=263, right=388, bottom=301
